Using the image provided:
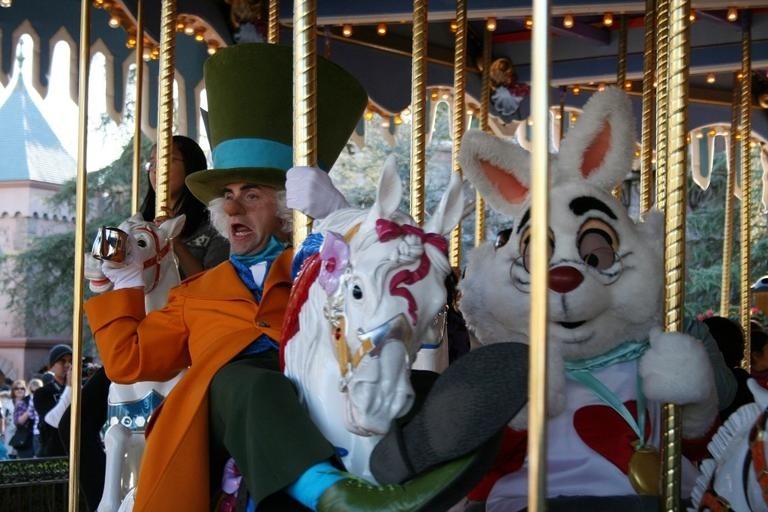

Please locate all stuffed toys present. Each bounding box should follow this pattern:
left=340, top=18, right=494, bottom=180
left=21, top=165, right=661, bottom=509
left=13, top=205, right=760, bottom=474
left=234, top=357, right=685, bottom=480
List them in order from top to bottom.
left=452, top=83, right=741, bottom=510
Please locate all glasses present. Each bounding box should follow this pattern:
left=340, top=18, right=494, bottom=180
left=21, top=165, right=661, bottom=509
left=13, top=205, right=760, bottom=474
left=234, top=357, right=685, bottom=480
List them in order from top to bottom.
left=14, top=386, right=24, bottom=391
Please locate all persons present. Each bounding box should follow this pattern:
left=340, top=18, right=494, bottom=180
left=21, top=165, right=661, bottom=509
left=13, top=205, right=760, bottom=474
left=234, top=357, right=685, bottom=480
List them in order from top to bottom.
left=78, top=134, right=530, bottom=511
left=139, top=135, right=233, bottom=281
left=700, top=314, right=764, bottom=424
left=739, top=326, right=768, bottom=398
left=0, top=340, right=107, bottom=464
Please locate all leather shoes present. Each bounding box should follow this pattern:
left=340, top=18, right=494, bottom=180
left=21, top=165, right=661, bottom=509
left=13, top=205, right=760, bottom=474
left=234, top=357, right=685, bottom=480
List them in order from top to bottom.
left=370, top=342, right=529, bottom=487
left=316, top=439, right=506, bottom=511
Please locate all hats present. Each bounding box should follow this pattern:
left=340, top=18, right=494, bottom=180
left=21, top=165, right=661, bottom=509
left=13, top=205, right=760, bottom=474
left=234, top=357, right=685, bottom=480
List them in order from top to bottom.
left=185, top=41, right=369, bottom=207
left=48, top=344, right=73, bottom=367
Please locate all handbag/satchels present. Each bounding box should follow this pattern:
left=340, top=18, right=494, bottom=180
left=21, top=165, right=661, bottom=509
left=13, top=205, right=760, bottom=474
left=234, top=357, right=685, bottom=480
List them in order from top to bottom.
left=9, top=430, right=33, bottom=451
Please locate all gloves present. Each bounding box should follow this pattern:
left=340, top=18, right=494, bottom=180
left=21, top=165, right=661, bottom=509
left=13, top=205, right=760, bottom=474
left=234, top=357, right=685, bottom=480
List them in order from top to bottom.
left=100, top=220, right=148, bottom=290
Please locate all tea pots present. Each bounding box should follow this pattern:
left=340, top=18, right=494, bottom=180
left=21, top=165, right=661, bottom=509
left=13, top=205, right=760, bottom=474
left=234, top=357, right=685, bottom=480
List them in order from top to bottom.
left=91, top=224, right=129, bottom=266
